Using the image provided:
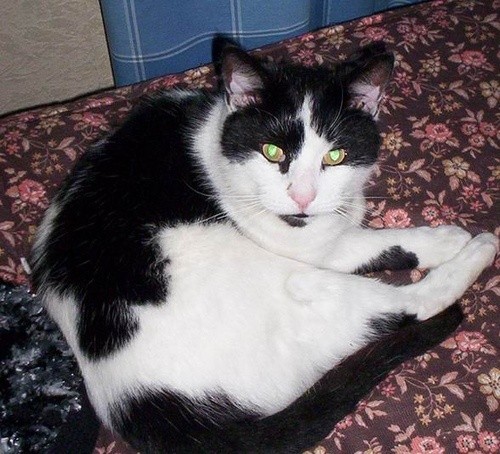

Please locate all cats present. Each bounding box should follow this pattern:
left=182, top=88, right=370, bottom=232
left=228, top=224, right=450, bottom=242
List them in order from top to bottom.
left=17, top=31, right=500, bottom=454
